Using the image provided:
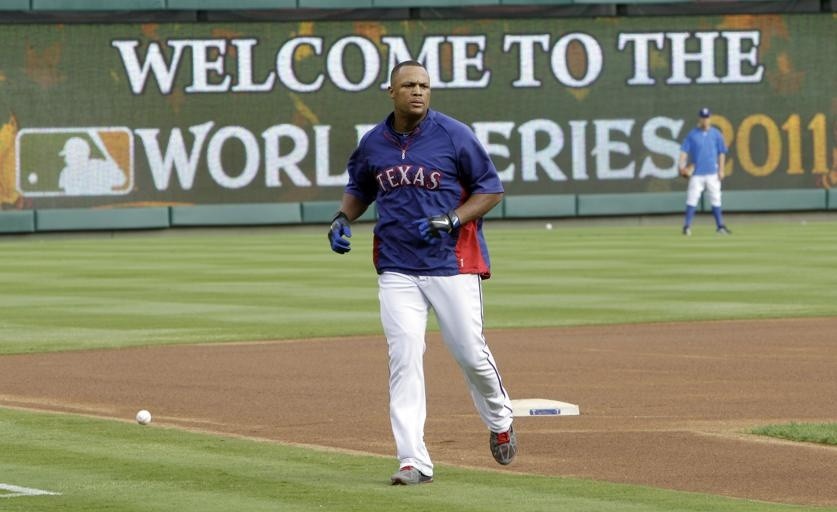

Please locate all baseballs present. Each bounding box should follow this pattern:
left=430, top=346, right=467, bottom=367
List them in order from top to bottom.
left=136, top=410, right=151, bottom=425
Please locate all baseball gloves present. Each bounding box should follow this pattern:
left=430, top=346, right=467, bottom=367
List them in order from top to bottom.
left=677, top=163, right=693, bottom=179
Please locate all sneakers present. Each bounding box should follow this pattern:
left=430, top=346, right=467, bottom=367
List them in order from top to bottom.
left=683, top=224, right=731, bottom=235
left=489, top=424, right=517, bottom=466
left=390, top=466, right=434, bottom=486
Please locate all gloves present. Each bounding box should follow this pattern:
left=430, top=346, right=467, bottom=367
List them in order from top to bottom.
left=412, top=210, right=461, bottom=245
left=328, top=211, right=353, bottom=255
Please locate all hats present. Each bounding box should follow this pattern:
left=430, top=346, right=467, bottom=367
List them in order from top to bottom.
left=698, top=108, right=712, bottom=118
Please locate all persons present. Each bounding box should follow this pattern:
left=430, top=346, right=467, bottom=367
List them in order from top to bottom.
left=325, top=60, right=518, bottom=486
left=677, top=107, right=732, bottom=236
